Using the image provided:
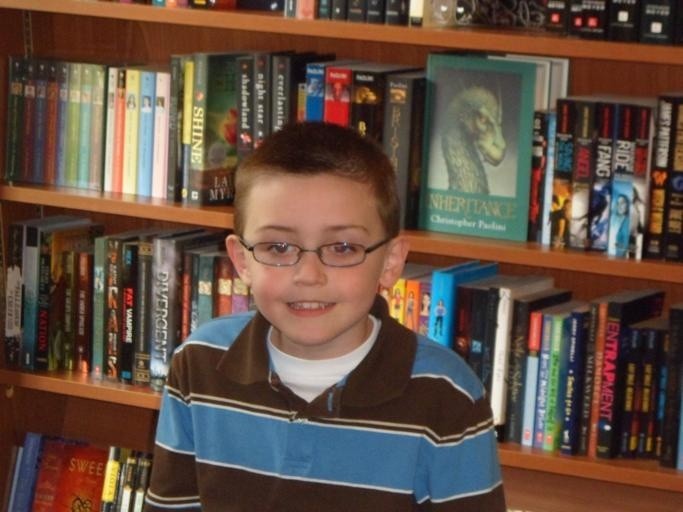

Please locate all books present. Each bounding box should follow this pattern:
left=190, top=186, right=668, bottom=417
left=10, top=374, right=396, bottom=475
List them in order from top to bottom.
left=0, top=432, right=154, bottom=512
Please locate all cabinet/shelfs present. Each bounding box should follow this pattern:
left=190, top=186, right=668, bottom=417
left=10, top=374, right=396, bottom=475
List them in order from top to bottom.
left=0, top=0, right=683, bottom=511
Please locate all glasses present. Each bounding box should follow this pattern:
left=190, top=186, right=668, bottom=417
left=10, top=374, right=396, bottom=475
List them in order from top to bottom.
left=240, top=236, right=395, bottom=268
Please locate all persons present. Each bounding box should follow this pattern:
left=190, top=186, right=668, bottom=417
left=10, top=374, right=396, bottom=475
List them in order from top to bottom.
left=140, top=120, right=508, bottom=512
